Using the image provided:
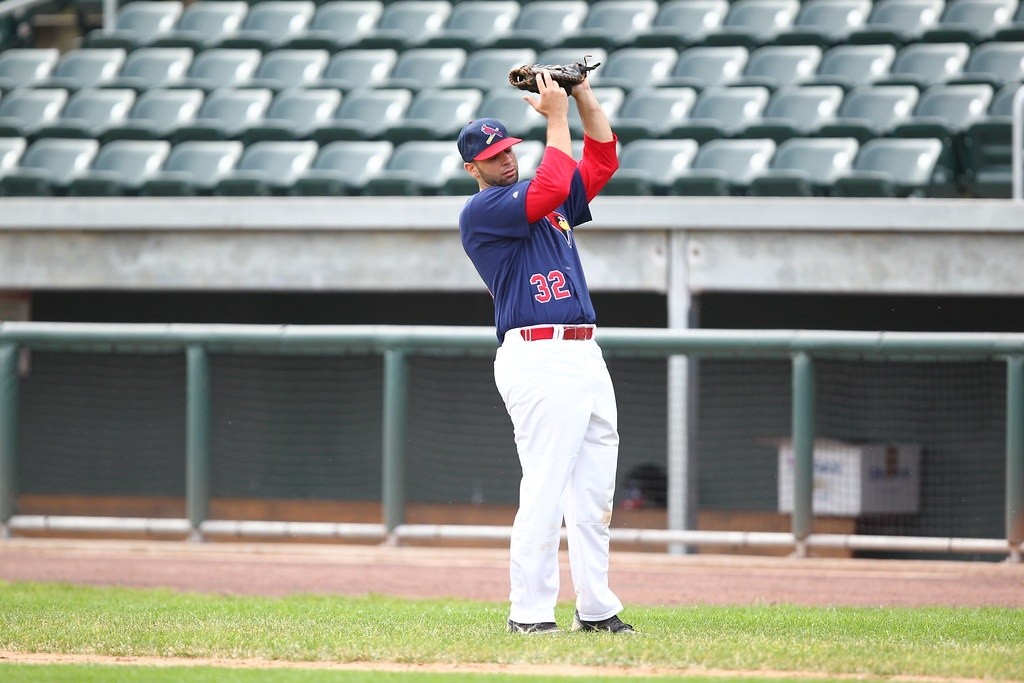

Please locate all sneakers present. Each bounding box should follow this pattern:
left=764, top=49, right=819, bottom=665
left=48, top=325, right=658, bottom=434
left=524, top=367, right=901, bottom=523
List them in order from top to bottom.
left=571, top=610, right=635, bottom=634
left=507, top=619, right=563, bottom=635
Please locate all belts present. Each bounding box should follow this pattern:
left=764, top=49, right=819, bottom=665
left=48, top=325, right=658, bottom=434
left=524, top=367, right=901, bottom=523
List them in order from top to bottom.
left=520, top=326, right=593, bottom=341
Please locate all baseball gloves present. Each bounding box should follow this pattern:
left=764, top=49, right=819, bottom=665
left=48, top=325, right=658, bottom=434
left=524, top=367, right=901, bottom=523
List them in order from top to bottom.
left=508, top=55, right=601, bottom=96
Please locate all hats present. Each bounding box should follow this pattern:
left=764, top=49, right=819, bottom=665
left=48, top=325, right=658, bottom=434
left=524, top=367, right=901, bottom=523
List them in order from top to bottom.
left=457, top=118, right=523, bottom=162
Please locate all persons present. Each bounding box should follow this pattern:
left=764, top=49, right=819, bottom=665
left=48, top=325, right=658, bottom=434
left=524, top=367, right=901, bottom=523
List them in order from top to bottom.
left=457, top=69, right=633, bottom=635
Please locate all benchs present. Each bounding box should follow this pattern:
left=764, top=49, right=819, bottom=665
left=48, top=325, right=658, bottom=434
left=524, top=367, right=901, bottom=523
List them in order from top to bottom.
left=14, top=493, right=906, bottom=560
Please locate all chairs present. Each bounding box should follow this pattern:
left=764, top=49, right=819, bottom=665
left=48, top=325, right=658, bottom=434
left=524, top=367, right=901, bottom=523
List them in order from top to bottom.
left=2, top=1, right=1023, bottom=197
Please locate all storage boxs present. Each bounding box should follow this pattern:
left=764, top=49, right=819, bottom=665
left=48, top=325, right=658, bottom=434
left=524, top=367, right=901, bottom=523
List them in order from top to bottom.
left=761, top=435, right=922, bottom=516
